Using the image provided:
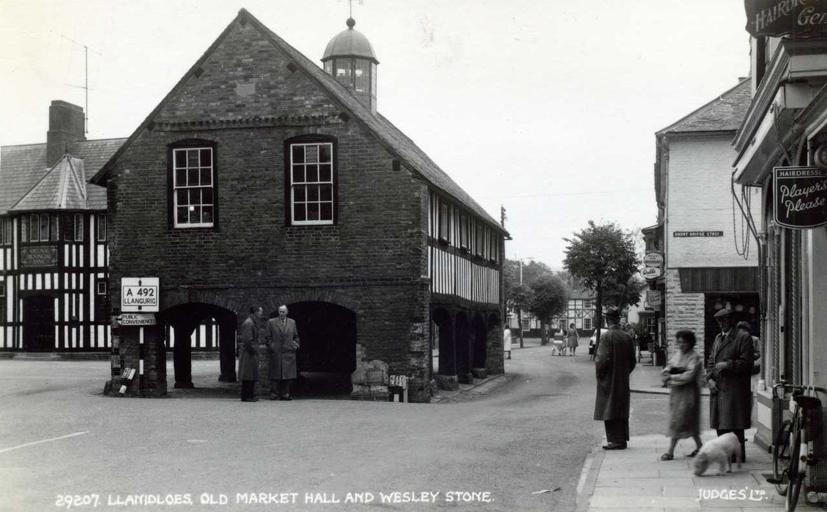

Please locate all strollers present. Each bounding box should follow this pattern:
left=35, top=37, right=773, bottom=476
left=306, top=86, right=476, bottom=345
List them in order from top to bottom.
left=551, top=333, right=567, bottom=356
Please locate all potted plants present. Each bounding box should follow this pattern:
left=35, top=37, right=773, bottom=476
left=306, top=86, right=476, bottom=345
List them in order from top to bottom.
left=634, top=324, right=652, bottom=350
left=654, top=343, right=668, bottom=366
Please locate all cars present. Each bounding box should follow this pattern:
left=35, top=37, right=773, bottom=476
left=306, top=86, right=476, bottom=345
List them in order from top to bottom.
left=587, top=328, right=608, bottom=355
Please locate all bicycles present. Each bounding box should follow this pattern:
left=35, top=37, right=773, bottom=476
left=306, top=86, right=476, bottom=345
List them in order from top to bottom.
left=769, top=381, right=827, bottom=511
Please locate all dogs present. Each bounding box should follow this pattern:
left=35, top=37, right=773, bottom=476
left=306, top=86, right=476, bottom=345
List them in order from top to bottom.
left=693, top=431, right=742, bottom=476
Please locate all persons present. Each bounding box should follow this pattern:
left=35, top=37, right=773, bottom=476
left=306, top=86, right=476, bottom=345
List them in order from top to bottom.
left=237, top=304, right=263, bottom=402
left=263, top=304, right=301, bottom=400
left=705, top=308, right=751, bottom=465
left=592, top=309, right=636, bottom=451
left=735, top=320, right=760, bottom=429
left=617, top=321, right=640, bottom=341
left=553, top=322, right=578, bottom=356
left=503, top=324, right=511, bottom=359
left=658, top=329, right=703, bottom=461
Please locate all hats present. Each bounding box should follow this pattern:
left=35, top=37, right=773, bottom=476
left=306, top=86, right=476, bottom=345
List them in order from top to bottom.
left=602, top=305, right=624, bottom=318
left=714, top=309, right=732, bottom=317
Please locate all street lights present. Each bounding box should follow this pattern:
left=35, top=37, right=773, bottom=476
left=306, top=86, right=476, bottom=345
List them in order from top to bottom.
left=518, top=255, right=539, bottom=350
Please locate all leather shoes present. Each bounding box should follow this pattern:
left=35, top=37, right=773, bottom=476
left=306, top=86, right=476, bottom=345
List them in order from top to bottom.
left=241, top=397, right=258, bottom=402
left=603, top=443, right=627, bottom=449
left=661, top=453, right=674, bottom=460
